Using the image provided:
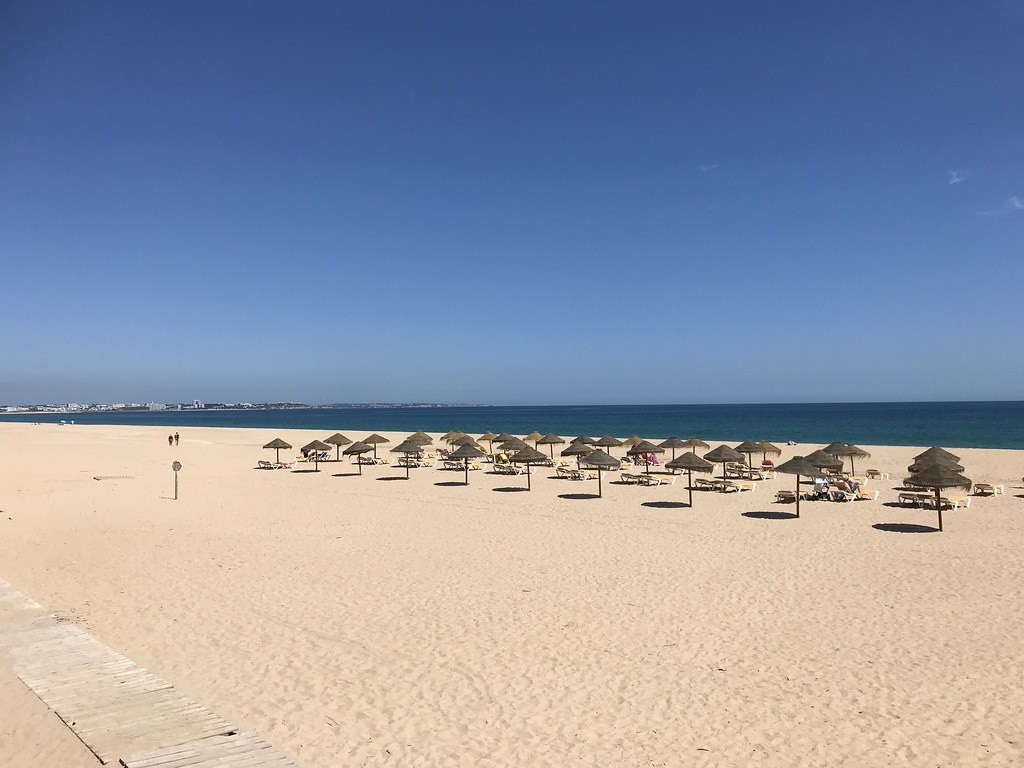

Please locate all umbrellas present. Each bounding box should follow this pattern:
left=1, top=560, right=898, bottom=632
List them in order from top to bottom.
left=361, top=431, right=463, bottom=479
left=451, top=431, right=543, bottom=492
left=536, top=432, right=623, bottom=499
left=263, top=433, right=374, bottom=475
left=623, top=434, right=664, bottom=475
left=658, top=436, right=692, bottom=474
left=685, top=436, right=710, bottom=454
left=733, top=439, right=766, bottom=479
left=758, top=440, right=781, bottom=462
left=822, top=441, right=859, bottom=481
left=703, top=444, right=745, bottom=488
left=845, top=444, right=871, bottom=477
left=913, top=446, right=961, bottom=463
left=803, top=449, right=843, bottom=472
left=664, top=452, right=713, bottom=506
left=908, top=452, right=966, bottom=473
left=769, top=456, right=826, bottom=518
left=904, top=463, right=972, bottom=533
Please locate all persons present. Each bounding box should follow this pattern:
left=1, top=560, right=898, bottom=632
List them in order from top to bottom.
left=174, top=431, right=180, bottom=445
left=168, top=434, right=173, bottom=445
left=648, top=453, right=657, bottom=464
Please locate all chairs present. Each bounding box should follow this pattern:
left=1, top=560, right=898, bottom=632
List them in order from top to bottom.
left=357, top=447, right=972, bottom=512
left=258, top=451, right=331, bottom=471
left=973, top=483, right=1004, bottom=497
